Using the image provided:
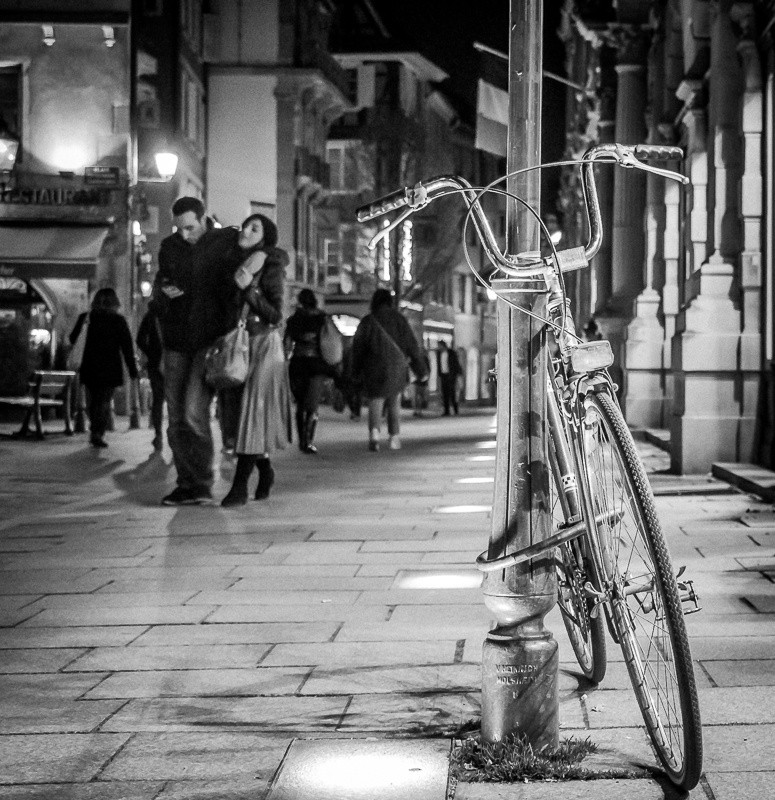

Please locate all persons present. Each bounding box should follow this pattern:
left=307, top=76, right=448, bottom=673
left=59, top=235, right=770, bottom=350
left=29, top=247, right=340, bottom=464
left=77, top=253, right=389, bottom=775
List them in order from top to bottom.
left=134, top=196, right=289, bottom=507
left=68, top=287, right=141, bottom=447
left=353, top=288, right=431, bottom=451
left=283, top=290, right=345, bottom=454
left=339, top=336, right=363, bottom=420
left=413, top=341, right=462, bottom=421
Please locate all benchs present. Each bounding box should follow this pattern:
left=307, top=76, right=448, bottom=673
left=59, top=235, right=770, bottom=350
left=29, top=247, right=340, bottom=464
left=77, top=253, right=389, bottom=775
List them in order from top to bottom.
left=0, top=371, right=80, bottom=442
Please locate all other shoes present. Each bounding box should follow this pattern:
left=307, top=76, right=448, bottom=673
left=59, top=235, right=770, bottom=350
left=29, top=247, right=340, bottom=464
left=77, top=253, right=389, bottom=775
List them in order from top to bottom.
left=91, top=436, right=108, bottom=448
left=151, top=437, right=163, bottom=450
left=387, top=440, right=401, bottom=450
left=369, top=441, right=381, bottom=451
left=162, top=487, right=193, bottom=505
left=194, top=487, right=213, bottom=504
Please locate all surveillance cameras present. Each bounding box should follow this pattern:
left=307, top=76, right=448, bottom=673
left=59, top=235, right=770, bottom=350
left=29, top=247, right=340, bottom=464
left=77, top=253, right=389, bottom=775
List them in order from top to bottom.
left=140, top=99, right=160, bottom=128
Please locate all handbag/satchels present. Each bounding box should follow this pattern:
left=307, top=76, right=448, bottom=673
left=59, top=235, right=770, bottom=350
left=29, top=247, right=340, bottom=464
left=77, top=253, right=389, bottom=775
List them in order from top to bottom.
left=65, top=312, right=90, bottom=371
left=320, top=316, right=342, bottom=366
left=203, top=325, right=249, bottom=387
left=407, top=365, right=418, bottom=383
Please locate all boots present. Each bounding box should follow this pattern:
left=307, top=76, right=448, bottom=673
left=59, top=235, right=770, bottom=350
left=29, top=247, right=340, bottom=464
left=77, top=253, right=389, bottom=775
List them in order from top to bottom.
left=300, top=430, right=318, bottom=453
left=254, top=471, right=274, bottom=499
left=221, top=473, right=247, bottom=506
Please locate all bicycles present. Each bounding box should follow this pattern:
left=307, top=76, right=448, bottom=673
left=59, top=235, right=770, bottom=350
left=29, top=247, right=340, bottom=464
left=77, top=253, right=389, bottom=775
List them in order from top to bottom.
left=354, top=145, right=705, bottom=793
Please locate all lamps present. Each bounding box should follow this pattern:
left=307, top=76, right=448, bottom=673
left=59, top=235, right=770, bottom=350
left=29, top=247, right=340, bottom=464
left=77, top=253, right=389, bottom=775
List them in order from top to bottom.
left=137, top=152, right=179, bottom=183
